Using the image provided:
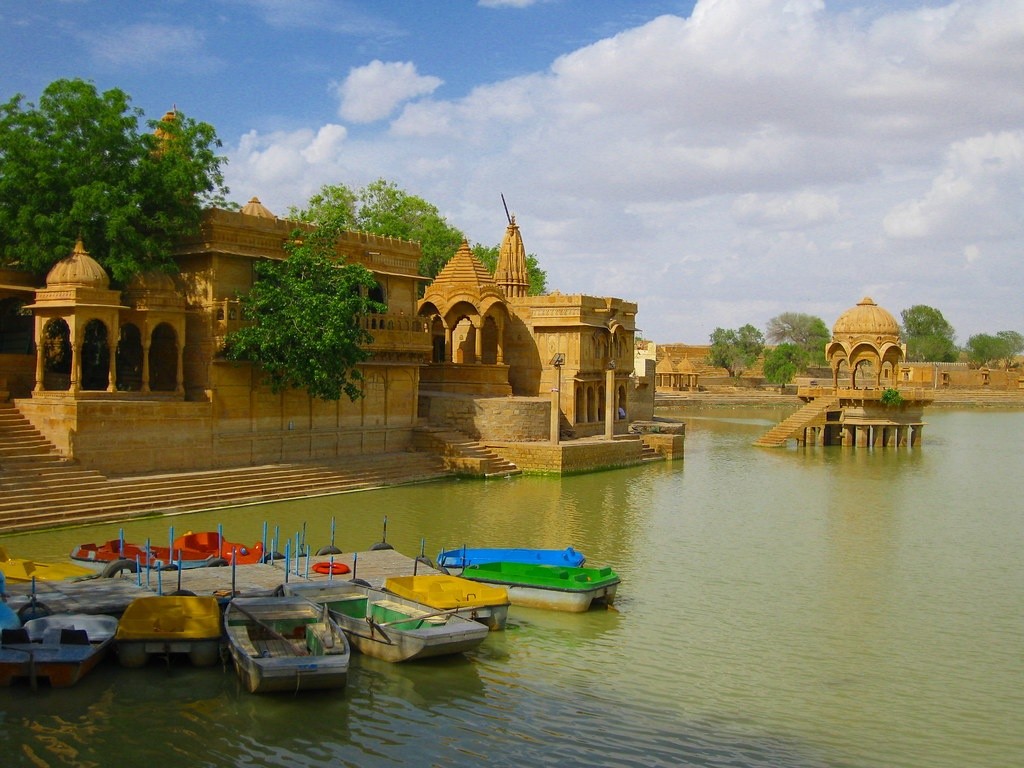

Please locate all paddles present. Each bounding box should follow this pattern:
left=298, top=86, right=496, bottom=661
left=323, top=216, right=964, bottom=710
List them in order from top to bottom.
left=22, top=561, right=50, bottom=576
left=229, top=600, right=309, bottom=656
left=322, top=601, right=335, bottom=648
left=366, top=605, right=486, bottom=644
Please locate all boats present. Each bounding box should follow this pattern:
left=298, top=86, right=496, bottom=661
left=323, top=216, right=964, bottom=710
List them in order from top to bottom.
left=173, top=532, right=263, bottom=566
left=69, top=537, right=217, bottom=574
left=0, top=545, right=101, bottom=589
left=436, top=547, right=586, bottom=577
left=462, top=561, right=621, bottom=613
left=381, top=573, right=512, bottom=631
left=282, top=581, right=490, bottom=663
left=113, top=596, right=221, bottom=673
left=224, top=596, right=350, bottom=699
left=0, top=613, right=119, bottom=691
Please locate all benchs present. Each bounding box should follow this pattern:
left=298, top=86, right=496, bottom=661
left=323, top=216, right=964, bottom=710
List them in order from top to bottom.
left=305, top=592, right=368, bottom=618
left=370, top=600, right=446, bottom=631
left=228, top=611, right=318, bottom=640
left=306, top=623, right=345, bottom=656
left=229, top=626, right=259, bottom=657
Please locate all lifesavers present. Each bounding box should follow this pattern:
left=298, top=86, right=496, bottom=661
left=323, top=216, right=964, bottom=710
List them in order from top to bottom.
left=369, top=542, right=394, bottom=551
left=315, top=545, right=342, bottom=556
left=258, top=552, right=287, bottom=563
left=295, top=553, right=306, bottom=558
left=415, top=555, right=434, bottom=567
left=102, top=557, right=142, bottom=580
left=207, top=557, right=229, bottom=567
left=312, top=562, right=351, bottom=574
left=154, top=564, right=180, bottom=571
left=436, top=566, right=451, bottom=575
left=349, top=578, right=372, bottom=587
left=170, top=589, right=197, bottom=598
left=17, top=602, right=53, bottom=621
